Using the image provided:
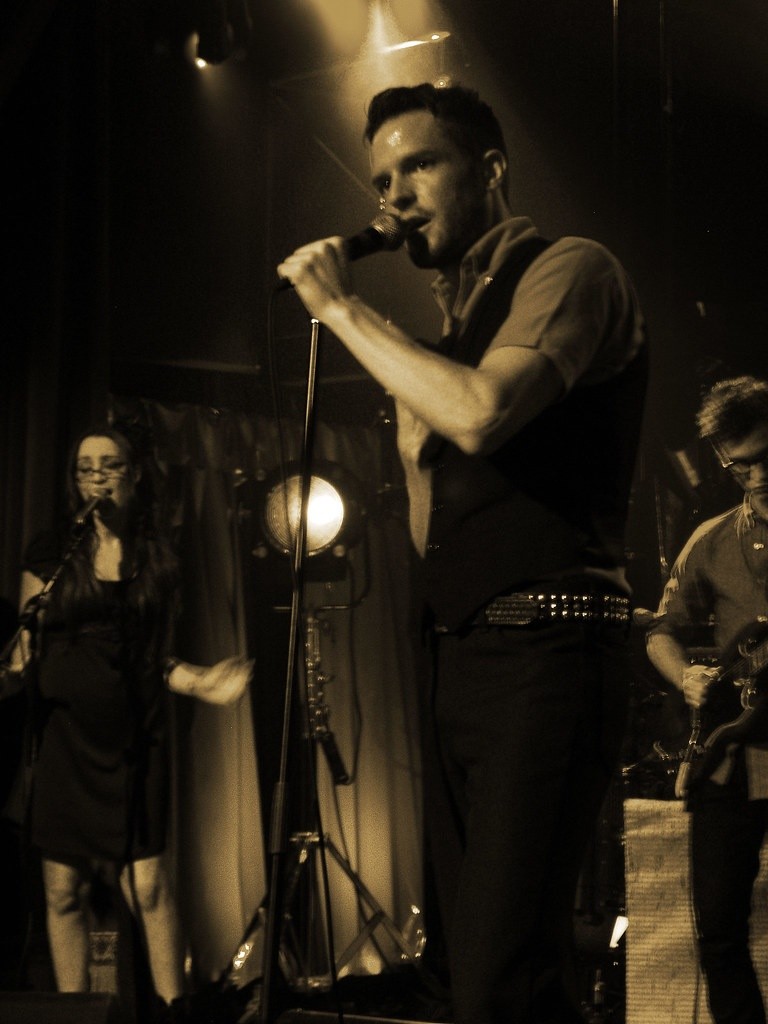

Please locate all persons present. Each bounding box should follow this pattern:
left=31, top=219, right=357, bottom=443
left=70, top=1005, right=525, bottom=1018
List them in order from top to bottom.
left=274, top=86, right=644, bottom=1024
left=643, top=377, right=768, bottom=1024
left=2, top=426, right=196, bottom=1009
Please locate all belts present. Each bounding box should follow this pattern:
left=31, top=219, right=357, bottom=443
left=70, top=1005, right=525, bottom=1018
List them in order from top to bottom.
left=432, top=590, right=631, bottom=631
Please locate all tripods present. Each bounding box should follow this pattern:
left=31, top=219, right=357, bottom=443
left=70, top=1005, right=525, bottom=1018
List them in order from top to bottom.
left=218, top=604, right=424, bottom=1007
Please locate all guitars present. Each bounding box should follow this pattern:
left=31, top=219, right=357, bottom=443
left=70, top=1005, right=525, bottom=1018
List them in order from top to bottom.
left=696, top=612, right=768, bottom=751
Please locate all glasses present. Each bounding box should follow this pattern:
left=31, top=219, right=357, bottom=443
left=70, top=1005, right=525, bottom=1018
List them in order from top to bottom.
left=710, top=443, right=768, bottom=474
left=73, top=464, right=130, bottom=481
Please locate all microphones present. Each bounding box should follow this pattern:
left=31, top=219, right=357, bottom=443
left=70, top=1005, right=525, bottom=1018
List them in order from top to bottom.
left=276, top=213, right=406, bottom=291
left=76, top=489, right=106, bottom=525
